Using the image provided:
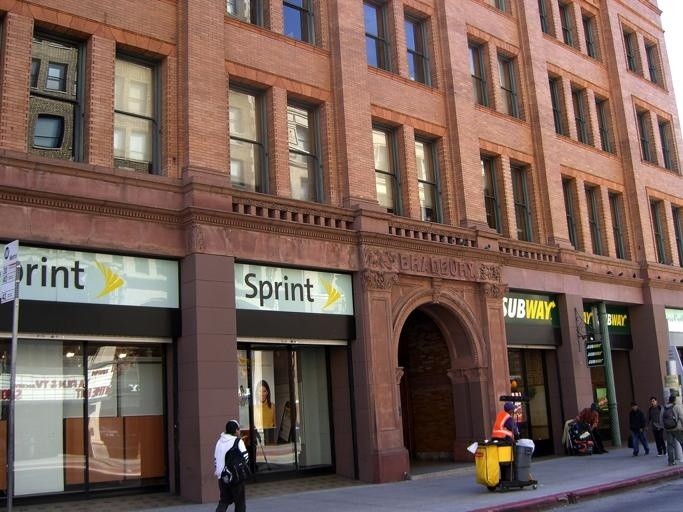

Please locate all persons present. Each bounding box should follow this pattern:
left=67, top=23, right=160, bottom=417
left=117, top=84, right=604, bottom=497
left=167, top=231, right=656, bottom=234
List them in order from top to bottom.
left=253, top=380, right=276, bottom=428
left=659, top=395, right=683, bottom=466
left=644, top=397, right=667, bottom=456
left=629, top=402, right=649, bottom=456
left=492, top=403, right=520, bottom=481
left=576, top=403, right=608, bottom=454
left=215, top=420, right=249, bottom=512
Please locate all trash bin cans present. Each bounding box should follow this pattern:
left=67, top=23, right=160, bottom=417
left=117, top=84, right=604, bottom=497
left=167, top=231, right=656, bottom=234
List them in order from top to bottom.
left=471, top=441, right=533, bottom=487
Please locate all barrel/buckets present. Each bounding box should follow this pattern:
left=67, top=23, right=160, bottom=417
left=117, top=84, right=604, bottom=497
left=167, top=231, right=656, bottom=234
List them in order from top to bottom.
left=514, top=445, right=533, bottom=482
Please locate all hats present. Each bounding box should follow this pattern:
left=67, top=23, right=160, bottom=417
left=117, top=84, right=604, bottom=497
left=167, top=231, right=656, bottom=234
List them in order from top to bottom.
left=630, top=401, right=639, bottom=405
left=504, top=403, right=517, bottom=411
left=591, top=403, right=600, bottom=410
left=226, top=420, right=240, bottom=434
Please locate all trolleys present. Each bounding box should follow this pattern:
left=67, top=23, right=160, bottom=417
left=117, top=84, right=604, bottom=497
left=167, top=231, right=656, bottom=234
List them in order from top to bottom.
left=487, top=442, right=537, bottom=493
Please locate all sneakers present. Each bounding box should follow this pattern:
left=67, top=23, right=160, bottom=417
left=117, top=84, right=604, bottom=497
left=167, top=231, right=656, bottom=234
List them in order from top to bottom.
left=633, top=446, right=666, bottom=457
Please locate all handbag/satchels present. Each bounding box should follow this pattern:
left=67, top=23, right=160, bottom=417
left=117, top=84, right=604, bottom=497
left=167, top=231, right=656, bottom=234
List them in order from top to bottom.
left=627, top=436, right=634, bottom=448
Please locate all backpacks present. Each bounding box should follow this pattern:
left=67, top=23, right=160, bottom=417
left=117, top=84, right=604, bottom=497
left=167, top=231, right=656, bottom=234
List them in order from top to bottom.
left=662, top=403, right=678, bottom=429
left=221, top=437, right=251, bottom=486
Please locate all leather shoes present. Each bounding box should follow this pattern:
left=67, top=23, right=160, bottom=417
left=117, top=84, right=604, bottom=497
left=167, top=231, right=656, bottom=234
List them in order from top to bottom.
left=600, top=448, right=609, bottom=453
